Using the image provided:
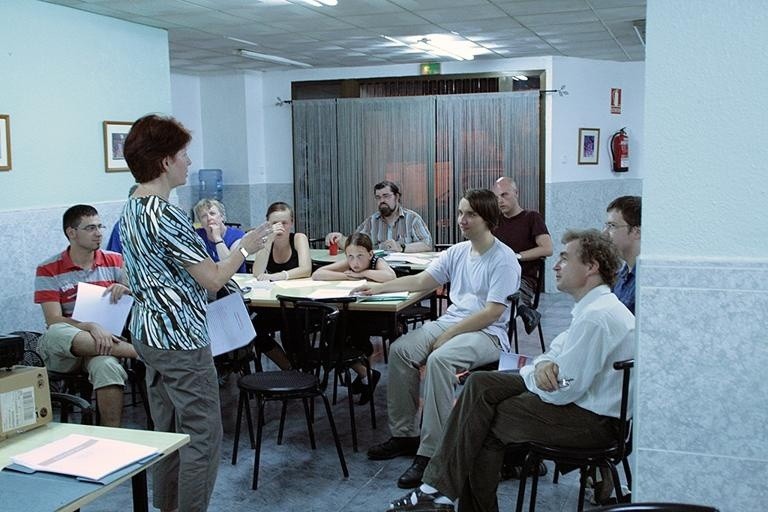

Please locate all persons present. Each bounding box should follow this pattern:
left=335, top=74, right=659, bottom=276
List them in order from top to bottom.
left=119, top=112, right=273, bottom=512
left=489, top=177, right=553, bottom=336
left=194, top=181, right=432, bottom=406
left=106, top=185, right=136, bottom=251
left=349, top=189, right=522, bottom=489
left=601, top=196, right=641, bottom=317
left=35, top=204, right=140, bottom=427
left=387, top=227, right=641, bottom=512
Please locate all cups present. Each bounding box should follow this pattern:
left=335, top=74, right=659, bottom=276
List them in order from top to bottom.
left=329, top=237, right=338, bottom=258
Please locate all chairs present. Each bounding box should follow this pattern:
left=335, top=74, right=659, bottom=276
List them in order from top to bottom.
left=0, top=223, right=722, bottom=511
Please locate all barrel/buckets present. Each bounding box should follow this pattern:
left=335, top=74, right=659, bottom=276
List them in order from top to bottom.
left=198, top=168, right=223, bottom=202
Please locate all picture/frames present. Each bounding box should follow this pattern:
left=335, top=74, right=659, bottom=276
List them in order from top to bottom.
left=0, top=114, right=12, bottom=171
left=103, top=120, right=136, bottom=173
left=577, top=127, right=600, bottom=165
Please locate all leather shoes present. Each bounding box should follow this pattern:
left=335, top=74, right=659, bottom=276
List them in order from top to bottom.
left=521, top=307, right=541, bottom=335
left=351, top=360, right=381, bottom=405
left=367, top=437, right=431, bottom=489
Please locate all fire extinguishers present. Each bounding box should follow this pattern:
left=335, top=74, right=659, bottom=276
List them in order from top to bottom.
left=608, top=127, right=629, bottom=172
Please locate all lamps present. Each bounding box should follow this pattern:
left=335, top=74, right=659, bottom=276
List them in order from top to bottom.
left=417, top=37, right=475, bottom=62
left=239, top=49, right=312, bottom=68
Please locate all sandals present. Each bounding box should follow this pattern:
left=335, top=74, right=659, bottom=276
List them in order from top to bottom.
left=385, top=487, right=455, bottom=512
